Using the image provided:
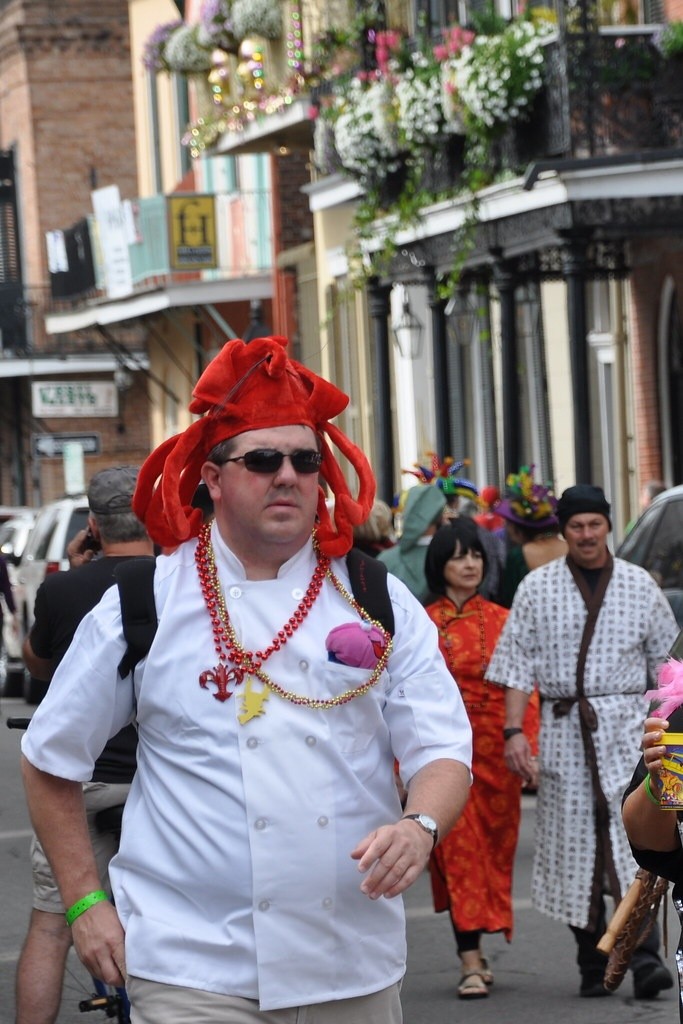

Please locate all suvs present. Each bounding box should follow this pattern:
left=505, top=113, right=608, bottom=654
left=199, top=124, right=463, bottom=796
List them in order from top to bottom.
left=612, top=483, right=683, bottom=634
left=0, top=493, right=90, bottom=706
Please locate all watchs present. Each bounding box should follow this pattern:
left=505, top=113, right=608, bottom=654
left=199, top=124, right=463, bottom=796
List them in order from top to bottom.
left=502, top=727, right=523, bottom=741
left=400, top=814, right=438, bottom=848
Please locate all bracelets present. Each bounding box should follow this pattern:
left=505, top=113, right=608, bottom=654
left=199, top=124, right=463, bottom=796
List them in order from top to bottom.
left=644, top=773, right=660, bottom=806
left=65, top=889, right=107, bottom=928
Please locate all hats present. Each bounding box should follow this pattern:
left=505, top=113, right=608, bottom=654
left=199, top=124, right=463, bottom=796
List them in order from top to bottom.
left=131, top=334, right=377, bottom=558
left=403, top=450, right=482, bottom=506
left=89, top=463, right=146, bottom=515
left=498, top=463, right=561, bottom=530
left=560, top=484, right=613, bottom=538
left=400, top=485, right=449, bottom=554
left=482, top=486, right=502, bottom=509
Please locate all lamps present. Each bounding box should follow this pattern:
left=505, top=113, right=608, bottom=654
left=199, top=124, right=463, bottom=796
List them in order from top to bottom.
left=394, top=279, right=422, bottom=356
left=444, top=286, right=501, bottom=347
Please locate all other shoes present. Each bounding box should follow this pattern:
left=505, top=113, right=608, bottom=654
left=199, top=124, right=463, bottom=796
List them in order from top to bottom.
left=633, top=963, right=674, bottom=1000
left=579, top=972, right=612, bottom=998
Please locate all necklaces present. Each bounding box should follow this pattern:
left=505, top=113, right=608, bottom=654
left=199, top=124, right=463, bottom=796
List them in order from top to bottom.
left=195, top=517, right=392, bottom=710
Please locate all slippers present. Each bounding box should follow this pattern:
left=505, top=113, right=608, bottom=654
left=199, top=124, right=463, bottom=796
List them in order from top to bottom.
left=456, top=957, right=494, bottom=998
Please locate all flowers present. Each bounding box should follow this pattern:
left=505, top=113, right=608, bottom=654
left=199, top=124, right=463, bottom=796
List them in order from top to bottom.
left=144, top=0, right=285, bottom=75
left=311, top=0, right=595, bottom=331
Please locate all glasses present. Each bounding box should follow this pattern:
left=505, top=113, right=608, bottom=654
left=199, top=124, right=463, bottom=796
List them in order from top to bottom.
left=223, top=448, right=324, bottom=475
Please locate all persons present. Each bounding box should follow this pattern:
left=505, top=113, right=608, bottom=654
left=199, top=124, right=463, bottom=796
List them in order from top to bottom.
left=20, top=336, right=473, bottom=1024
left=325, top=453, right=571, bottom=609
left=0, top=465, right=216, bottom=1024
left=484, top=483, right=681, bottom=1003
left=394, top=524, right=542, bottom=998
left=621, top=630, right=683, bottom=1024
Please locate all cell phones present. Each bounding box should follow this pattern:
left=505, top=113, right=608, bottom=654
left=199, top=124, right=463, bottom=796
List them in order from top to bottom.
left=86, top=528, right=99, bottom=555
